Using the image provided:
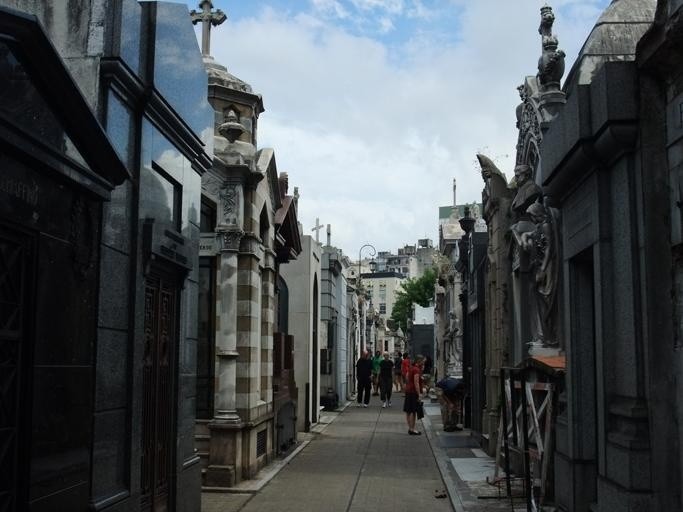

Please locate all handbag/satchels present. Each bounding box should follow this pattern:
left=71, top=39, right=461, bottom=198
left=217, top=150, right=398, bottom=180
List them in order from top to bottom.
left=415, top=401, right=424, bottom=420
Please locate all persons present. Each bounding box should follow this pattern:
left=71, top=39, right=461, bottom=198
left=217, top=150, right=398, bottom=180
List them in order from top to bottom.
left=510, top=163, right=541, bottom=209
left=514, top=200, right=560, bottom=347
left=447, top=310, right=465, bottom=367
left=356, top=350, right=373, bottom=408
left=393, top=350, right=432, bottom=394
left=372, top=351, right=382, bottom=396
left=379, top=351, right=394, bottom=408
left=403, top=353, right=427, bottom=435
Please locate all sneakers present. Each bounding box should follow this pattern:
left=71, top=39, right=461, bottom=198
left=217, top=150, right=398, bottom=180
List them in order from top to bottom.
left=355, top=400, right=393, bottom=408
left=444, top=425, right=462, bottom=433
left=407, top=429, right=421, bottom=435
